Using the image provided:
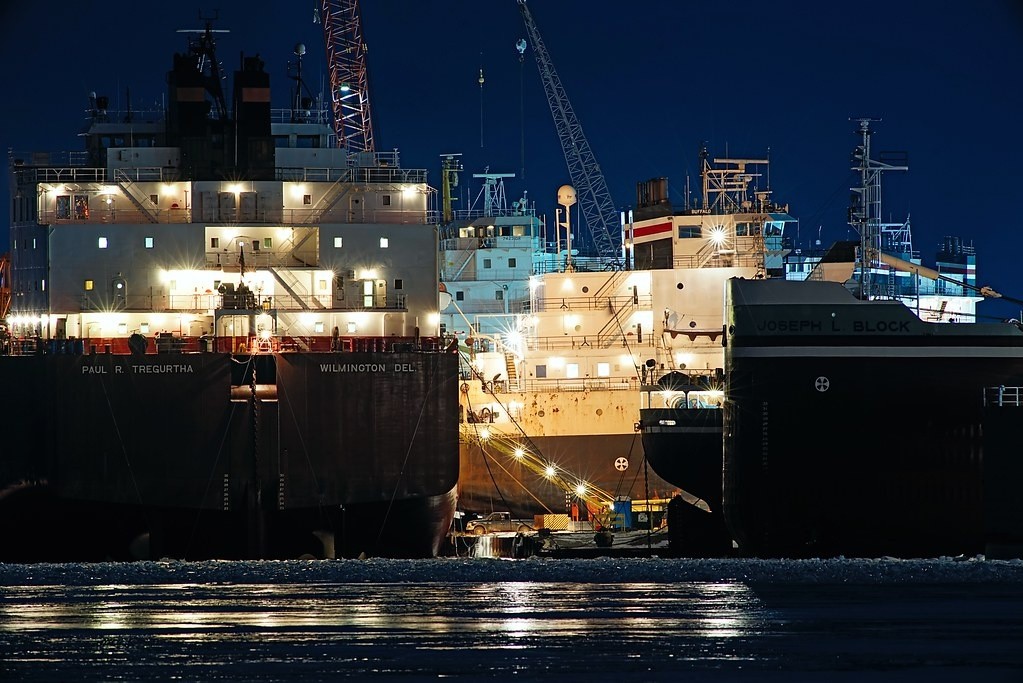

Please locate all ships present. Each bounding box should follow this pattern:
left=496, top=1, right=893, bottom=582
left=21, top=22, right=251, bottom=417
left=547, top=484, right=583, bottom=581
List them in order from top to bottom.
left=0, top=0, right=1022, bottom=564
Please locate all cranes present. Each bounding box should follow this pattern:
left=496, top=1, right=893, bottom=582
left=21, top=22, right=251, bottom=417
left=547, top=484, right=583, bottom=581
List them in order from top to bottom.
left=316, top=0, right=380, bottom=159
left=517, top=0, right=628, bottom=266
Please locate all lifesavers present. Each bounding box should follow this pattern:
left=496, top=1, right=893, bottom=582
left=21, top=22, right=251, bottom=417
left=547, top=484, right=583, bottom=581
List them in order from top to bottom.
left=460, top=383, right=470, bottom=393
